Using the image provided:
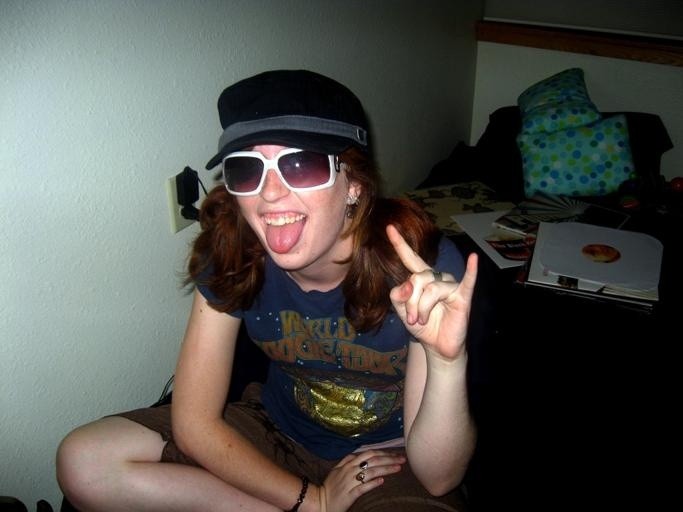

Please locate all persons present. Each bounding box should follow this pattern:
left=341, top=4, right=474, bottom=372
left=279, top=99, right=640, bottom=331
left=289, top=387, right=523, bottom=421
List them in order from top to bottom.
left=56, top=73, right=480, bottom=512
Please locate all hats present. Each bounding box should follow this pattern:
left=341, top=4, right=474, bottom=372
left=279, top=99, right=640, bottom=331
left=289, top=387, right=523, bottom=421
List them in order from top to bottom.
left=206, top=69, right=373, bottom=170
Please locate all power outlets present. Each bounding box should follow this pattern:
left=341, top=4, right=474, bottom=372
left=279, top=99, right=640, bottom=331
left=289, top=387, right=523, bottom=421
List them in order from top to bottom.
left=167, top=170, right=200, bottom=236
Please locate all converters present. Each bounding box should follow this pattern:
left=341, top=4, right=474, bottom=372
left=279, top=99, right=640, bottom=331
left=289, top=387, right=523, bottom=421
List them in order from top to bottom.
left=176, top=169, right=199, bottom=206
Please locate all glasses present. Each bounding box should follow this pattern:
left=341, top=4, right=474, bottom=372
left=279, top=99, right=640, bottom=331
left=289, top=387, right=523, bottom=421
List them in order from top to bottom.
left=222, top=148, right=349, bottom=196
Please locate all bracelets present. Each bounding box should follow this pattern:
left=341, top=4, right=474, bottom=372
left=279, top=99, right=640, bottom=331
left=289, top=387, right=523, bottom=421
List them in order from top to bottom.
left=284, top=474, right=308, bottom=512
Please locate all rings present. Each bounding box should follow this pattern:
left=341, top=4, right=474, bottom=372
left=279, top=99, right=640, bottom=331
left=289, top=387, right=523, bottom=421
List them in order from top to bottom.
left=433, top=271, right=443, bottom=282
left=359, top=460, right=369, bottom=470
left=356, top=472, right=366, bottom=484
